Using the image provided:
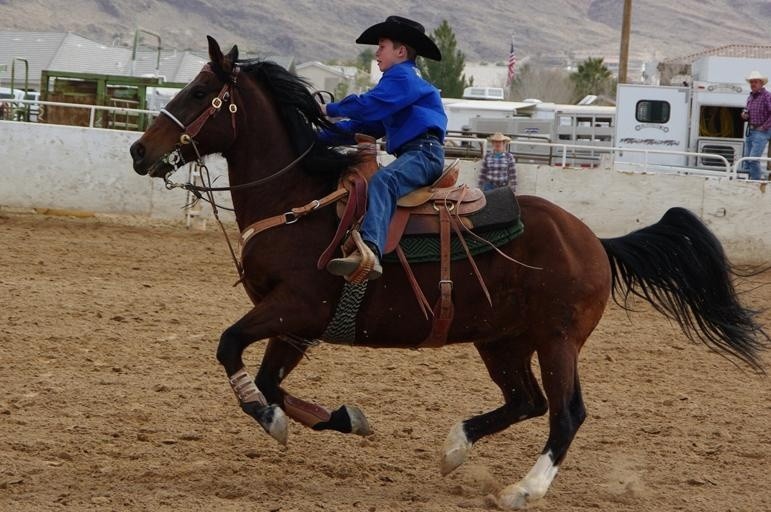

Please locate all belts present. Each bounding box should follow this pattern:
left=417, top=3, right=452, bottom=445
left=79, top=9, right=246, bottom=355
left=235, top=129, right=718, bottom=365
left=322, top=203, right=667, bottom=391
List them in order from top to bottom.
left=748, top=125, right=756, bottom=130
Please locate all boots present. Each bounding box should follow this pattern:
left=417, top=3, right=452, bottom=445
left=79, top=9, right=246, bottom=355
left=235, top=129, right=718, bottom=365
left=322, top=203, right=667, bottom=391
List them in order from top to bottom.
left=326, top=241, right=383, bottom=281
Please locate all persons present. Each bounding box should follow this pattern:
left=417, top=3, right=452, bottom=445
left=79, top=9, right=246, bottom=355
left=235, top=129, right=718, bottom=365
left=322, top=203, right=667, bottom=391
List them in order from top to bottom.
left=314, top=15, right=449, bottom=283
left=741, top=70, right=771, bottom=180
left=478, top=131, right=518, bottom=196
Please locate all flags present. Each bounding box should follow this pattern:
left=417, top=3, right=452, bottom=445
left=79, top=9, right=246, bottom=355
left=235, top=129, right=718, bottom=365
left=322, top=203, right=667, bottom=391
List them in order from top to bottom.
left=505, top=40, right=516, bottom=88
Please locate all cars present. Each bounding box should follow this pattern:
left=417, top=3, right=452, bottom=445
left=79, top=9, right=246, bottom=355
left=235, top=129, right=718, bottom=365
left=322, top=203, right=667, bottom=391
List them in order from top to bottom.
left=1, top=87, right=24, bottom=121
left=20, top=91, right=41, bottom=122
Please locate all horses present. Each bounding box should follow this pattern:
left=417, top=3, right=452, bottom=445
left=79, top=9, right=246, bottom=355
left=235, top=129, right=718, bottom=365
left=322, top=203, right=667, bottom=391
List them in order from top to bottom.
left=130, top=35, right=771, bottom=509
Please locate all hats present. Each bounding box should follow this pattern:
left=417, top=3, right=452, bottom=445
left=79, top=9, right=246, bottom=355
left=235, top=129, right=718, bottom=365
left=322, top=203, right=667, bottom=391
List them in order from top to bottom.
left=356, top=16, right=441, bottom=61
left=745, top=71, right=768, bottom=84
left=487, top=132, right=510, bottom=142
